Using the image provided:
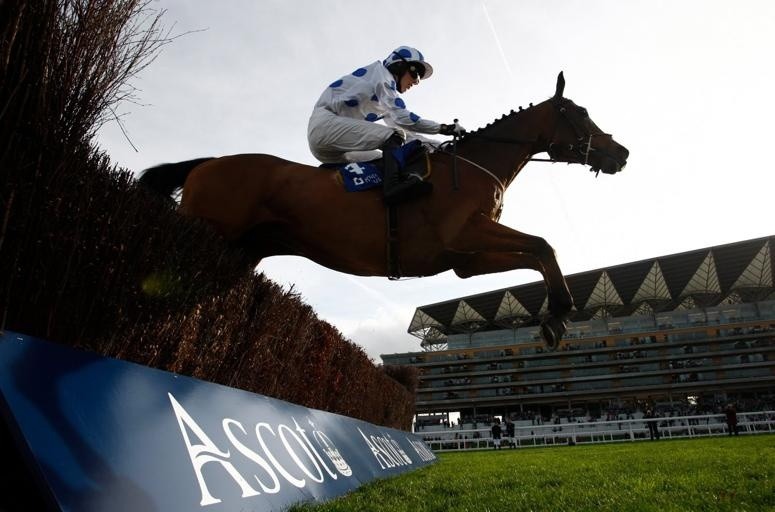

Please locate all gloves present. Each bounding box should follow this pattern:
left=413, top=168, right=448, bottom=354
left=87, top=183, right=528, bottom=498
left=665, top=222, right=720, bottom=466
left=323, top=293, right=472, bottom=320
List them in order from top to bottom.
left=441, top=123, right=465, bottom=135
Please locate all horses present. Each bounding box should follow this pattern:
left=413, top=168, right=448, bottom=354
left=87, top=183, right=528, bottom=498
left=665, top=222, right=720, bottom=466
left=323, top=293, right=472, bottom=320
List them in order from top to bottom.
left=129, top=70, right=631, bottom=356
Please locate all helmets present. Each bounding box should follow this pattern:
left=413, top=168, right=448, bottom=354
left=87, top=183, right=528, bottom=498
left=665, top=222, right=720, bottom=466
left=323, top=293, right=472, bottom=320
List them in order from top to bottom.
left=384, top=46, right=433, bottom=79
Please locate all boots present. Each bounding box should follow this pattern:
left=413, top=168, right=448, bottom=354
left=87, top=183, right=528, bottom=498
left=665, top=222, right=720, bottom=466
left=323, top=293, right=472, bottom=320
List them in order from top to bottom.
left=382, top=133, right=433, bottom=201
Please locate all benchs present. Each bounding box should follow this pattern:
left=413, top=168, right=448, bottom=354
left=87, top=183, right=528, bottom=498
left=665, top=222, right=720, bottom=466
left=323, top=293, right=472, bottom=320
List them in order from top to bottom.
left=499, top=413, right=649, bottom=445
left=657, top=413, right=728, bottom=440
left=415, top=414, right=495, bottom=449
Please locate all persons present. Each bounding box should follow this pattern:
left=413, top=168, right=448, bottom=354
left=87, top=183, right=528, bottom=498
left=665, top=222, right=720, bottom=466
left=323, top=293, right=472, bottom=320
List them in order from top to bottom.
left=306, top=45, right=467, bottom=208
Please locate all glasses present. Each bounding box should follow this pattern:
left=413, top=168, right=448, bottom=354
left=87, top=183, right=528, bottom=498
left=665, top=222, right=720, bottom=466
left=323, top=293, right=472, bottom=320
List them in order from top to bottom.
left=392, top=51, right=421, bottom=80
